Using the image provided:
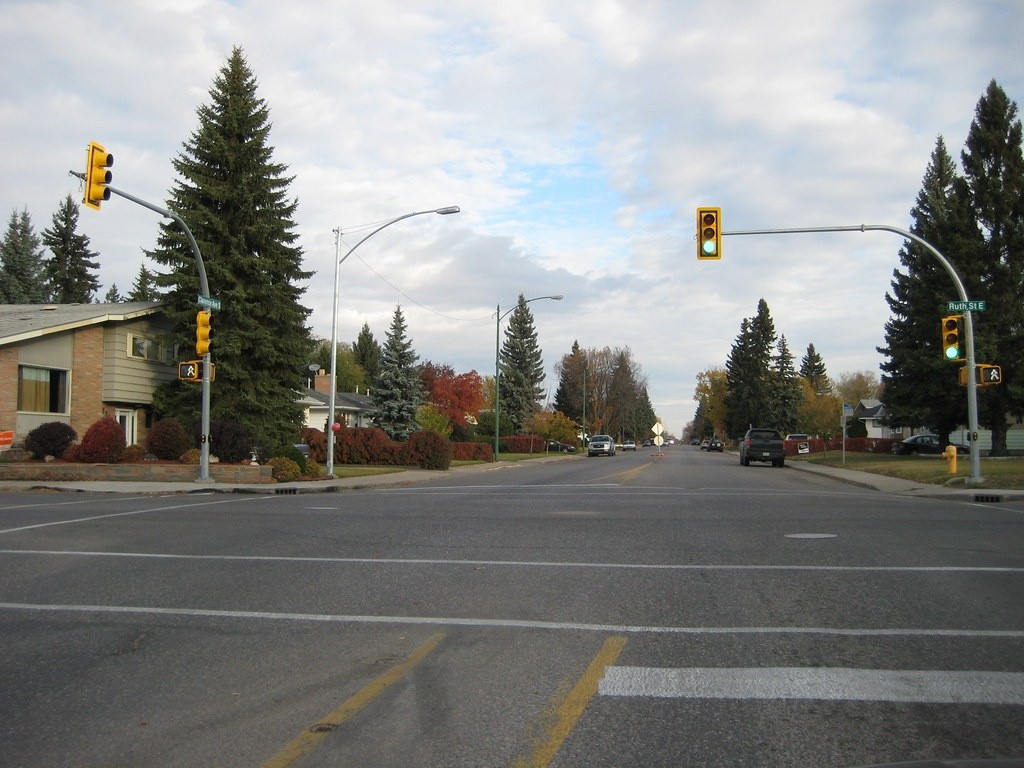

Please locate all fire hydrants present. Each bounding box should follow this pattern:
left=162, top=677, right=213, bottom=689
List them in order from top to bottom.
left=942, top=444, right=959, bottom=474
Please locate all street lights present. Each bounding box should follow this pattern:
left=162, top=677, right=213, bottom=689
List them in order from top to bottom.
left=327, top=203, right=463, bottom=476
left=494, top=296, right=563, bottom=463
left=583, top=365, right=619, bottom=452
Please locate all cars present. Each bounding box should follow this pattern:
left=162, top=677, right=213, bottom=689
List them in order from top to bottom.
left=738, top=429, right=785, bottom=467
left=890, top=434, right=969, bottom=455
left=588, top=435, right=616, bottom=457
left=641, top=436, right=725, bottom=452
left=544, top=439, right=575, bottom=453
left=622, top=440, right=636, bottom=450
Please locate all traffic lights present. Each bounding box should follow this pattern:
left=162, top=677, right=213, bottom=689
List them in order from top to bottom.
left=84, top=141, right=114, bottom=212
left=697, top=207, right=722, bottom=259
left=196, top=310, right=214, bottom=358
left=941, top=315, right=966, bottom=362
left=179, top=360, right=216, bottom=383
left=959, top=363, right=1005, bottom=386
